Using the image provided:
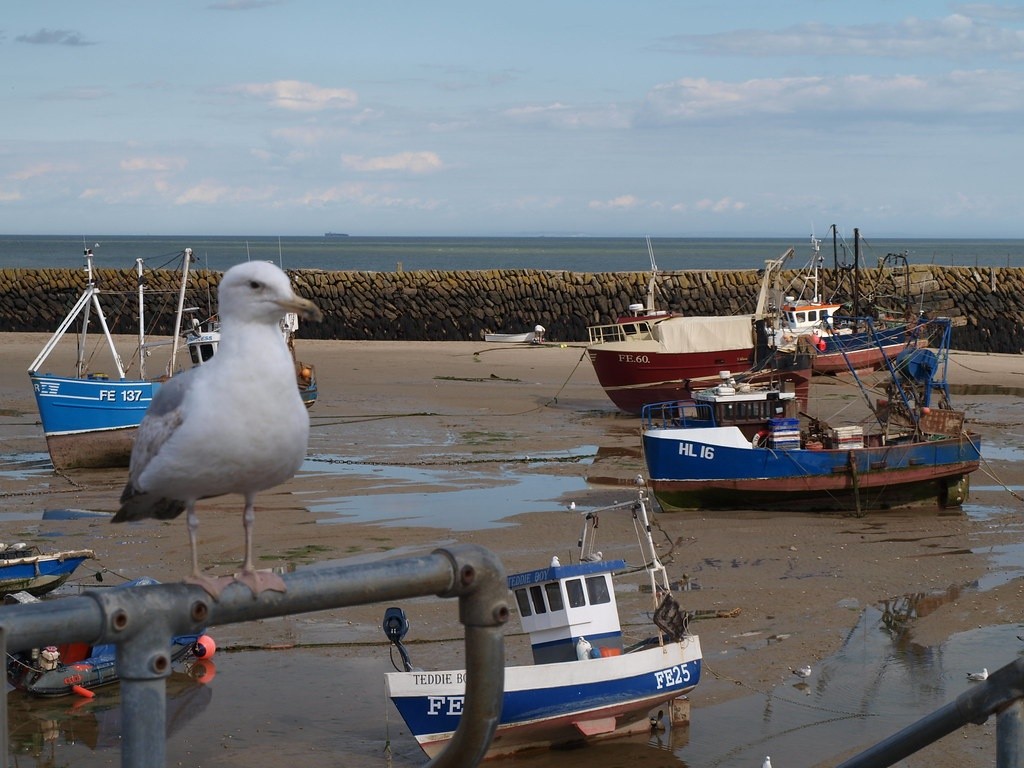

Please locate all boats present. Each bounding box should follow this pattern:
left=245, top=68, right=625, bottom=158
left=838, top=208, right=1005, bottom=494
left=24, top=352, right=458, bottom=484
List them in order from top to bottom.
left=584, top=224, right=981, bottom=516
left=28, top=249, right=317, bottom=472
left=485, top=331, right=536, bottom=343
left=383, top=473, right=703, bottom=760
left=0, top=543, right=216, bottom=699
left=7, top=669, right=212, bottom=768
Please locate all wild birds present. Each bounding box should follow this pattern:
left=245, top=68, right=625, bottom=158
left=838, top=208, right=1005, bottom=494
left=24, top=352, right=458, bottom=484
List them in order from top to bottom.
left=109, top=260, right=323, bottom=600
left=634, top=474, right=645, bottom=490
left=567, top=501, right=576, bottom=510
left=5, top=542, right=27, bottom=551
left=579, top=551, right=603, bottom=562
left=550, top=556, right=560, bottom=567
left=576, top=637, right=591, bottom=661
left=792, top=665, right=811, bottom=681
left=967, top=668, right=988, bottom=681
left=761, top=756, right=772, bottom=768
